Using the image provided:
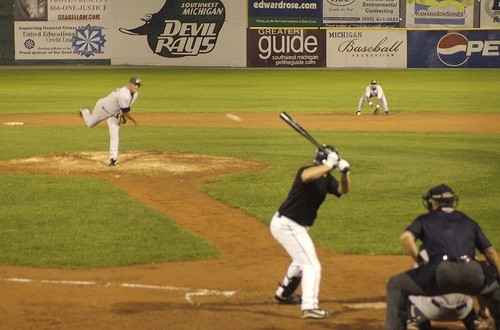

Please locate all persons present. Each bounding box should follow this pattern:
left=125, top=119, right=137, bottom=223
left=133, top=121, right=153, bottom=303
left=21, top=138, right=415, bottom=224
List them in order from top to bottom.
left=78, top=76, right=144, bottom=166
left=355, top=80, right=390, bottom=116
left=269, top=143, right=351, bottom=319
left=384, top=184, right=500, bottom=330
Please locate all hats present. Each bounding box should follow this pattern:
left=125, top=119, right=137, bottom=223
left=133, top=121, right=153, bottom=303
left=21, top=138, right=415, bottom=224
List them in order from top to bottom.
left=130, top=77, right=142, bottom=84
left=371, top=80, right=378, bottom=84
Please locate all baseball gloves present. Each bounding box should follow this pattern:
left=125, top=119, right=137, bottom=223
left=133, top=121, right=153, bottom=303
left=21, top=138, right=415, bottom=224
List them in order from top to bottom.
left=373, top=107, right=379, bottom=115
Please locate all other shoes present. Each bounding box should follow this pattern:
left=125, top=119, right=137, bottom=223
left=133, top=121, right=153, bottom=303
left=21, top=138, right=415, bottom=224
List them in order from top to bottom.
left=79, top=106, right=90, bottom=117
left=356, top=111, right=362, bottom=116
left=384, top=111, right=390, bottom=116
left=109, top=158, right=117, bottom=166
left=274, top=293, right=302, bottom=304
left=302, top=309, right=330, bottom=319
left=407, top=317, right=432, bottom=330
left=468, top=320, right=489, bottom=330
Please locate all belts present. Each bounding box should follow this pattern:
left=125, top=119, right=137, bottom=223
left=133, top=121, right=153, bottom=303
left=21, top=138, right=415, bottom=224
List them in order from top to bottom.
left=427, top=255, right=475, bottom=263
left=432, top=299, right=467, bottom=310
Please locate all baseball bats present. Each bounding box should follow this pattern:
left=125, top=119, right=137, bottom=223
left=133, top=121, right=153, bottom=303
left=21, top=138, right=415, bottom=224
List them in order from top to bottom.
left=279, top=112, right=350, bottom=175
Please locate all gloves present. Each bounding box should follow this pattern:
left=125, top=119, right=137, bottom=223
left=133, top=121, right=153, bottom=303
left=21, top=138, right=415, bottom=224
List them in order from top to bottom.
left=327, top=152, right=339, bottom=170
left=339, top=159, right=350, bottom=175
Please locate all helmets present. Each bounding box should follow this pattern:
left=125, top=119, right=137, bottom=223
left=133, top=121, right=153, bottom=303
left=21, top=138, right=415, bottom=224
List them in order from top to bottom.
left=313, top=145, right=340, bottom=173
left=426, top=184, right=455, bottom=211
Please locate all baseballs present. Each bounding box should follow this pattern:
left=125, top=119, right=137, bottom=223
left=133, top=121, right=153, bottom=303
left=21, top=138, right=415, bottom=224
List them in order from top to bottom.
left=227, top=114, right=240, bottom=121
